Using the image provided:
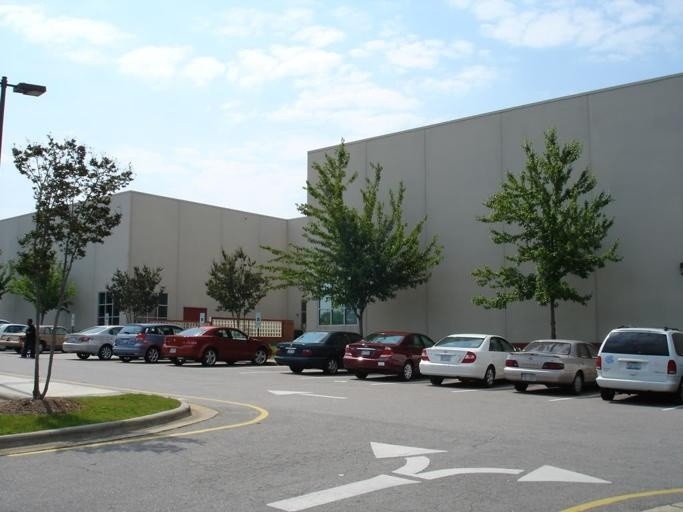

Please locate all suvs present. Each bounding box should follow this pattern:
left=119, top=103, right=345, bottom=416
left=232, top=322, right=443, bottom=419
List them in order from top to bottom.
left=593, top=326, right=683, bottom=404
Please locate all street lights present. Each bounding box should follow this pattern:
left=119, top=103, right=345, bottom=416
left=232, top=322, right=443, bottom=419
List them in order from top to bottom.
left=0, top=71, right=48, bottom=169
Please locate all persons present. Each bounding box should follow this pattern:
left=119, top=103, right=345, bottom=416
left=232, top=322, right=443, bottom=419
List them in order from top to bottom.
left=19, top=319, right=37, bottom=359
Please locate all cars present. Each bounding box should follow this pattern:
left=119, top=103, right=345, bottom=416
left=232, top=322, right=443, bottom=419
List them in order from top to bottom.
left=0, top=318, right=74, bottom=354
left=112, top=322, right=186, bottom=364
left=62, top=325, right=126, bottom=360
left=162, top=325, right=273, bottom=367
left=274, top=330, right=364, bottom=374
left=341, top=330, right=436, bottom=382
left=418, top=332, right=521, bottom=387
left=502, top=339, right=601, bottom=392
